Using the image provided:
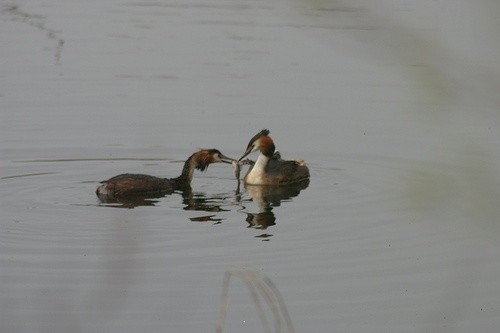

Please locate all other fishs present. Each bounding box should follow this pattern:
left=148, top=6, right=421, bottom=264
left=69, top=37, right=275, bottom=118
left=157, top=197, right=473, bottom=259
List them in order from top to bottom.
left=232, top=157, right=255, bottom=195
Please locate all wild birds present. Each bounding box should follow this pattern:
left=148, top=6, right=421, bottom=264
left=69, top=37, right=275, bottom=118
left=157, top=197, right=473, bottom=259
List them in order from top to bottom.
left=237, top=129, right=311, bottom=185
left=96, top=147, right=237, bottom=203
